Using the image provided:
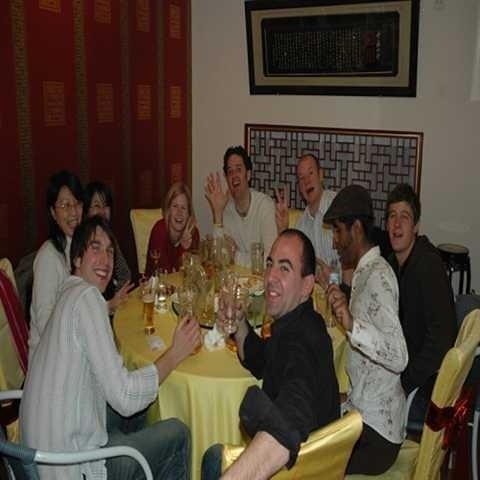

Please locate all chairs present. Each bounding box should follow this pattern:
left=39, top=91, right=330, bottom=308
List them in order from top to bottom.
left=130, top=208, right=163, bottom=274
left=0, top=257, right=29, bottom=390
left=406, top=295, right=480, bottom=479
left=346, top=308, right=480, bottom=480
left=0, top=389, right=153, bottom=480
left=221, top=409, right=363, bottom=480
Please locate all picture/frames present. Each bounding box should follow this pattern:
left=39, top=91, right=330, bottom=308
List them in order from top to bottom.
left=244, top=0, right=420, bottom=98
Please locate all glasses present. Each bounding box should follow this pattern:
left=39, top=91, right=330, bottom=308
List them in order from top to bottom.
left=53, top=199, right=84, bottom=212
left=88, top=205, right=112, bottom=214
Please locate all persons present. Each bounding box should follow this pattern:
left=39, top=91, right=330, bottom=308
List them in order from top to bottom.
left=205, top=146, right=278, bottom=268
left=275, top=153, right=341, bottom=278
left=26, top=171, right=135, bottom=357
left=83, top=176, right=132, bottom=292
left=144, top=182, right=200, bottom=281
left=314, top=184, right=410, bottom=474
left=387, top=184, right=456, bottom=391
left=18, top=214, right=201, bottom=480
left=201, top=229, right=342, bottom=480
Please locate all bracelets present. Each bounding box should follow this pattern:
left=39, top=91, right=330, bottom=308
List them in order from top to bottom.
left=212, top=224, right=225, bottom=228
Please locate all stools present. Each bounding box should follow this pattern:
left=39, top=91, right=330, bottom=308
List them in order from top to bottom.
left=437, top=243, right=471, bottom=300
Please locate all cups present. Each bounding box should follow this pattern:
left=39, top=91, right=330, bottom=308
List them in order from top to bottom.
left=137, top=233, right=337, bottom=353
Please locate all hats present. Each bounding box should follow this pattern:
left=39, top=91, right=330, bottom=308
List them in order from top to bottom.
left=324, top=186, right=373, bottom=224
